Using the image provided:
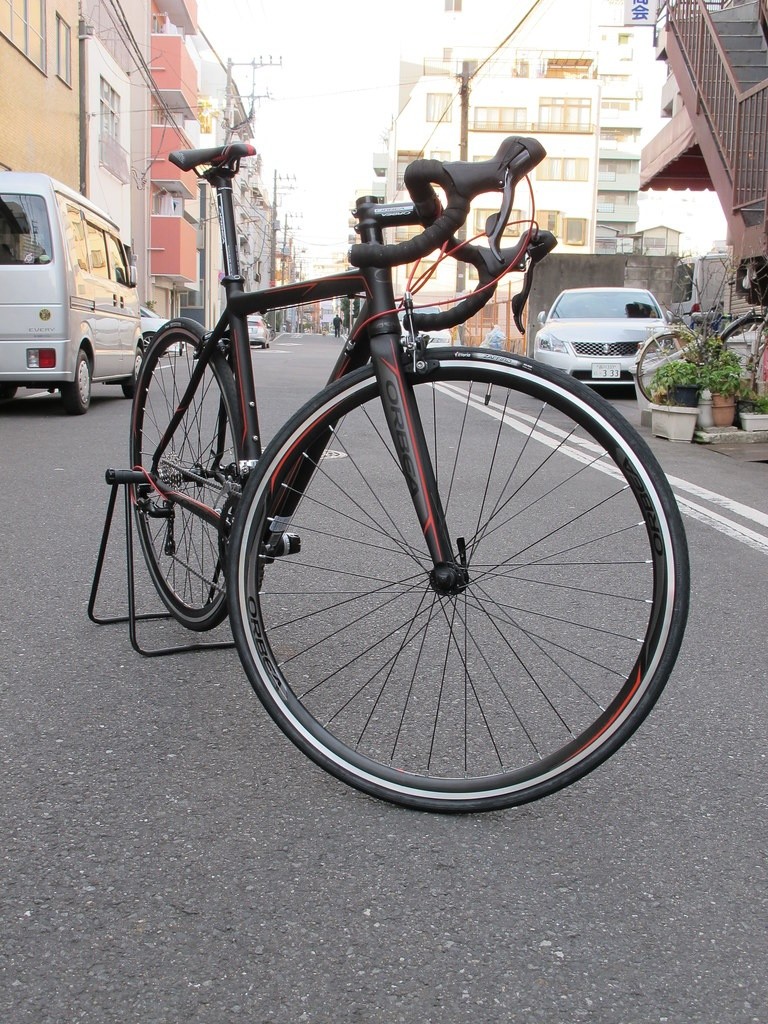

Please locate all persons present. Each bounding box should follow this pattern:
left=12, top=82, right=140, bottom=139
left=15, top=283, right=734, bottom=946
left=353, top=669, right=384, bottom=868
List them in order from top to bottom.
left=639, top=307, right=657, bottom=318
left=333, top=314, right=342, bottom=338
left=282, top=323, right=292, bottom=333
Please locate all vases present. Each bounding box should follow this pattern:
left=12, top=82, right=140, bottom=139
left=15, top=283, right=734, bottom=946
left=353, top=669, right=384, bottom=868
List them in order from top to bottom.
left=712, top=403, right=736, bottom=428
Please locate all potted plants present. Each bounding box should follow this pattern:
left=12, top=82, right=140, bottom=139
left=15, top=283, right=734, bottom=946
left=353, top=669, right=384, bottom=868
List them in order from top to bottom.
left=644, top=351, right=768, bottom=444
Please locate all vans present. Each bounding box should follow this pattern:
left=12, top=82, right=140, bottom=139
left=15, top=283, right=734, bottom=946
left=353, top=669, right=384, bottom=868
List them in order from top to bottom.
left=0, top=171, right=144, bottom=414
left=665, top=254, right=729, bottom=332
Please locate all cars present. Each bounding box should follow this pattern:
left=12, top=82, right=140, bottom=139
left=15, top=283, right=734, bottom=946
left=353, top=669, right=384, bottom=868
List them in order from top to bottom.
left=534, top=287, right=674, bottom=386
left=81, top=303, right=136, bottom=349
left=397, top=303, right=453, bottom=347
left=139, top=306, right=186, bottom=358
left=247, top=316, right=269, bottom=349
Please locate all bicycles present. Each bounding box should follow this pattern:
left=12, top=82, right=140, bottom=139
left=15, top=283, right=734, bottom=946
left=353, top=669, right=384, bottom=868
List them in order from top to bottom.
left=86, top=136, right=691, bottom=815
left=635, top=311, right=768, bottom=405
